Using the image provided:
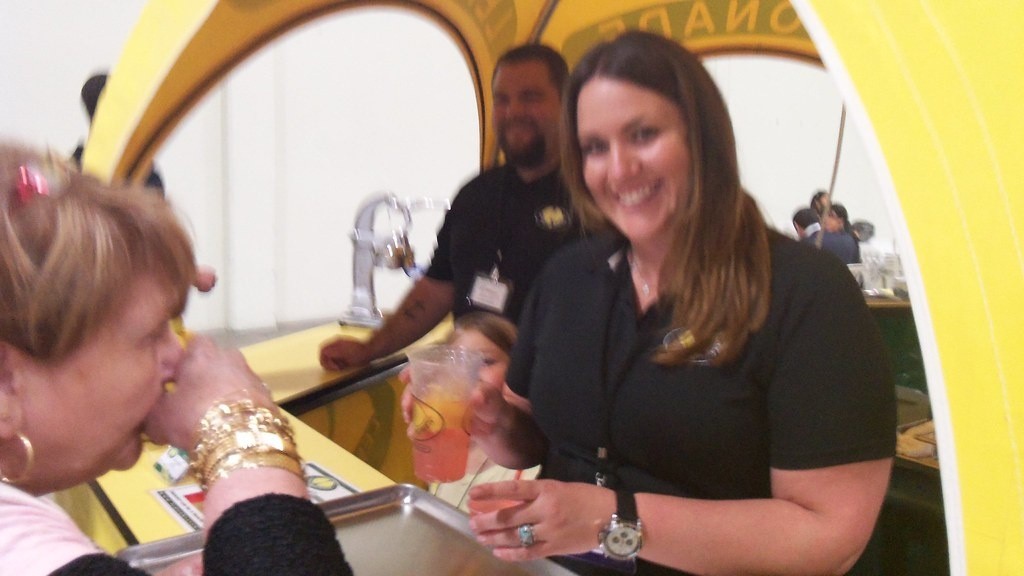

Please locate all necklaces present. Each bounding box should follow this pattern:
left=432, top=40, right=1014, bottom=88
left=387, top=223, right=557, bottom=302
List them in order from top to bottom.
left=633, top=255, right=658, bottom=296
left=432, top=454, right=489, bottom=510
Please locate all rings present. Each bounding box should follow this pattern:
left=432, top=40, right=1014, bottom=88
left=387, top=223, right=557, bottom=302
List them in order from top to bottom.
left=518, top=525, right=533, bottom=547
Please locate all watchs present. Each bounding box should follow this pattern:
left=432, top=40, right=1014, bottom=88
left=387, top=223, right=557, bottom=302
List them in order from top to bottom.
left=599, top=489, right=643, bottom=562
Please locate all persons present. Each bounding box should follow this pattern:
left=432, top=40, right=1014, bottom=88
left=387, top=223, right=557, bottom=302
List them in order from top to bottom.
left=398, top=29, right=895, bottom=576
left=320, top=42, right=588, bottom=369
left=67, top=73, right=218, bottom=292
left=0, top=136, right=358, bottom=576
left=793, top=192, right=874, bottom=264
left=426, top=309, right=541, bottom=518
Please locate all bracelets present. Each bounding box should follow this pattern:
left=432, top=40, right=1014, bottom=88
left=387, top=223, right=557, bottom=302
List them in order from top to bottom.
left=186, top=398, right=308, bottom=490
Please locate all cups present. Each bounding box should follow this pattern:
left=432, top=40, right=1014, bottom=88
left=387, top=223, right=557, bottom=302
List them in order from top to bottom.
left=864, top=249, right=882, bottom=296
left=882, top=253, right=902, bottom=290
left=847, top=264, right=861, bottom=286
left=895, top=278, right=908, bottom=299
left=408, top=345, right=484, bottom=482
left=468, top=499, right=530, bottom=536
